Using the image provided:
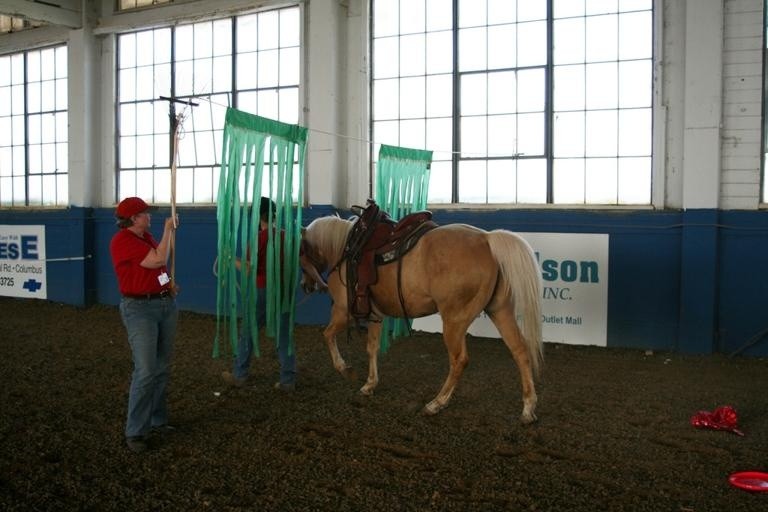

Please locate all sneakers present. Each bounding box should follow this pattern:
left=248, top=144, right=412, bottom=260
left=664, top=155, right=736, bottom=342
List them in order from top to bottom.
left=220, top=371, right=244, bottom=387
left=275, top=383, right=296, bottom=393
left=154, top=424, right=177, bottom=434
left=126, top=436, right=148, bottom=454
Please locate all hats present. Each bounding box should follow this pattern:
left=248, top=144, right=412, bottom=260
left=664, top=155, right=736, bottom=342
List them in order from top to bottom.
left=116, top=197, right=160, bottom=220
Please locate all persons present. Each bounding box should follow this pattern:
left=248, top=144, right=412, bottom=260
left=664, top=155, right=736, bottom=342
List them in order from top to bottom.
left=107, top=195, right=181, bottom=457
left=220, top=195, right=302, bottom=393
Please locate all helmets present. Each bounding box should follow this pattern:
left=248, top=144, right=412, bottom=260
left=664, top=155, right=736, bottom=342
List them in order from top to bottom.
left=249, top=196, right=277, bottom=214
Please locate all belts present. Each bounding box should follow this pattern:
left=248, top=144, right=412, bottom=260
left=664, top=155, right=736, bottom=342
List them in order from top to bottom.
left=122, top=292, right=170, bottom=300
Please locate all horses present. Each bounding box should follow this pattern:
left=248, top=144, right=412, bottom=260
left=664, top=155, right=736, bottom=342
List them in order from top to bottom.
left=293, top=209, right=547, bottom=426
